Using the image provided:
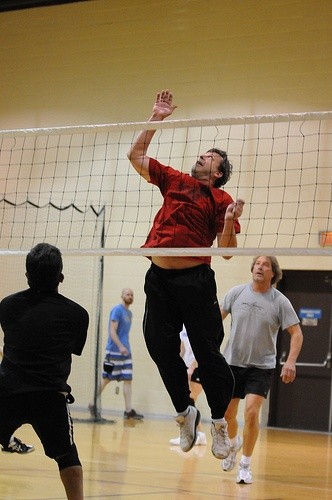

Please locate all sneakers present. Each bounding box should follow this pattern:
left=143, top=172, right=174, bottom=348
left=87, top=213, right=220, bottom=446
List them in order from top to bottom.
left=88, top=403, right=101, bottom=418
left=175, top=405, right=200, bottom=452
left=123, top=409, right=145, bottom=421
left=210, top=421, right=232, bottom=460
left=168, top=431, right=207, bottom=445
left=1, top=436, right=36, bottom=454
left=221, top=436, right=243, bottom=472
left=236, top=461, right=254, bottom=484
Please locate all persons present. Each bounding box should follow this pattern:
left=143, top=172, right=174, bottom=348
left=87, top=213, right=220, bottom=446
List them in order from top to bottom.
left=127, top=90, right=244, bottom=459
left=0, top=243, right=89, bottom=500
left=219, top=257, right=303, bottom=484
left=88, top=288, right=144, bottom=420
left=170, top=323, right=207, bottom=446
left=1, top=436, right=35, bottom=454
